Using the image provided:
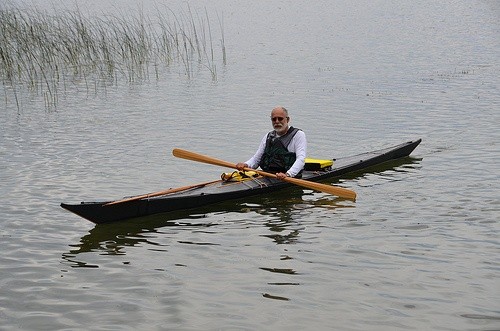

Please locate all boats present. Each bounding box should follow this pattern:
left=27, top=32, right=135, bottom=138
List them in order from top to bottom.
left=55, top=137, right=425, bottom=225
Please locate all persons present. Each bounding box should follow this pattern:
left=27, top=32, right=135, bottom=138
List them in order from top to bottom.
left=237, top=105, right=307, bottom=183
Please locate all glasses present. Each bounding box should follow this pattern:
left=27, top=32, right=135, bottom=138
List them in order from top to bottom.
left=272, top=117, right=286, bottom=121
